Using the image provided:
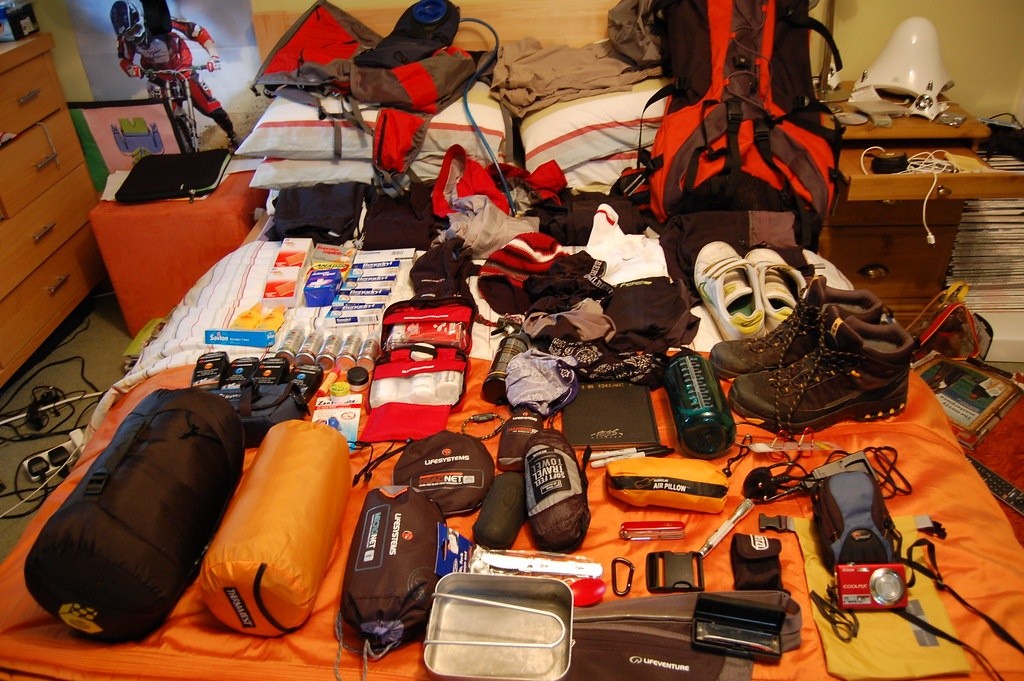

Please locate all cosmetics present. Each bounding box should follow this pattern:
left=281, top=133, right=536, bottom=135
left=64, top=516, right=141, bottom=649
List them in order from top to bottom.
left=331, top=367, right=370, bottom=404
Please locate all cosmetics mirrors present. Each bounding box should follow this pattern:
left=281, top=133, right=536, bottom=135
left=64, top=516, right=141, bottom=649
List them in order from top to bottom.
left=830, top=112, right=869, bottom=125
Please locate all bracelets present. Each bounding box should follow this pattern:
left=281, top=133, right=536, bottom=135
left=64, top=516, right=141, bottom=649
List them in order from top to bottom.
left=460, top=412, right=506, bottom=442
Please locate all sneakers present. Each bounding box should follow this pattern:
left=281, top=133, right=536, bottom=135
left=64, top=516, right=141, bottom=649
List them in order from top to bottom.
left=228, top=133, right=243, bottom=149
left=693, top=241, right=765, bottom=341
left=744, top=248, right=807, bottom=332
left=709, top=276, right=894, bottom=381
left=727, top=305, right=914, bottom=441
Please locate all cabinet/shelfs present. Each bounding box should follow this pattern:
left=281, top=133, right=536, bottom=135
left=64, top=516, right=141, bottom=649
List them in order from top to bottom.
left=0, top=33, right=111, bottom=405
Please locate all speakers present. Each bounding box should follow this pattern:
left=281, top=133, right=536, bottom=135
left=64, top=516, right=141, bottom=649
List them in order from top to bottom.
left=825, top=62, right=841, bottom=91
left=908, top=80, right=942, bottom=121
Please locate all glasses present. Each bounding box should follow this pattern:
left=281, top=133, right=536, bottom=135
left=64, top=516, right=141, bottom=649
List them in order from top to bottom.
left=123, top=22, right=145, bottom=41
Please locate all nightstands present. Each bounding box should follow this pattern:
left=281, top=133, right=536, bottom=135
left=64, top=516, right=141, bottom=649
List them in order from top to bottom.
left=815, top=80, right=1024, bottom=303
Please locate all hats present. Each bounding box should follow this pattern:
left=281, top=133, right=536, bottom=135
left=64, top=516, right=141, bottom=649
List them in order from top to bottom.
left=477, top=232, right=570, bottom=316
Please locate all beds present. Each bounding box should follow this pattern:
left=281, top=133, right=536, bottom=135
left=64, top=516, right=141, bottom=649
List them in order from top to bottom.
left=0, top=1, right=1022, bottom=681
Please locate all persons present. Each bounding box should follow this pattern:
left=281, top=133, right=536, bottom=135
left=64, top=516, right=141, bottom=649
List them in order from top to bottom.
left=109, top=0, right=244, bottom=152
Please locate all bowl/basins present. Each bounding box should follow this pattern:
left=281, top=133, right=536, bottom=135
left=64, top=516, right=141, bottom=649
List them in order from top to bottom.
left=423, top=571, right=574, bottom=681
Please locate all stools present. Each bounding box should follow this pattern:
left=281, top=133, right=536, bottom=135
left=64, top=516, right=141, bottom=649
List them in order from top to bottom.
left=91, top=160, right=273, bottom=339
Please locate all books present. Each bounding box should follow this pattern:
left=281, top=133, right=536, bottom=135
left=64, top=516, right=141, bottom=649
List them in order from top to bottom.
left=941, top=148, right=1024, bottom=313
left=903, top=352, right=1021, bottom=452
left=562, top=379, right=661, bottom=450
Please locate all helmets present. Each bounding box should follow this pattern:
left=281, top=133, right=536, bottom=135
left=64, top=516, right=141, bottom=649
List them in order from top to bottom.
left=110, top=0, right=140, bottom=41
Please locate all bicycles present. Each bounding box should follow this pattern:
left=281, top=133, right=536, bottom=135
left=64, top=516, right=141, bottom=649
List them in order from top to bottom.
left=141, top=63, right=208, bottom=156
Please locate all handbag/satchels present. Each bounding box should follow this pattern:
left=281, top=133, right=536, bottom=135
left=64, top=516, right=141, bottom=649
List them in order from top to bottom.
left=809, top=468, right=902, bottom=578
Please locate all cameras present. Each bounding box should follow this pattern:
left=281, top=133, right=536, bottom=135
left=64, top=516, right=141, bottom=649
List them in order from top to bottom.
left=834, top=561, right=907, bottom=611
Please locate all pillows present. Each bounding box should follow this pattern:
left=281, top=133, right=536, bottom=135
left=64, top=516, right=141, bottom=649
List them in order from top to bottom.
left=519, top=75, right=674, bottom=177
left=234, top=88, right=508, bottom=163
left=564, top=143, right=658, bottom=188
left=246, top=155, right=511, bottom=189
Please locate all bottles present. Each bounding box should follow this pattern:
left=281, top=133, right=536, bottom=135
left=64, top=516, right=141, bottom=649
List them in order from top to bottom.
left=481, top=330, right=529, bottom=404
left=664, top=348, right=738, bottom=460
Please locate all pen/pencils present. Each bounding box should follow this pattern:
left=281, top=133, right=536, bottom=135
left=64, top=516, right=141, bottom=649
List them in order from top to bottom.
left=581, top=445, right=675, bottom=469
left=698, top=498, right=755, bottom=558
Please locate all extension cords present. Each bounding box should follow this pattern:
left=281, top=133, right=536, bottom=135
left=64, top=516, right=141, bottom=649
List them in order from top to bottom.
left=22, top=440, right=81, bottom=482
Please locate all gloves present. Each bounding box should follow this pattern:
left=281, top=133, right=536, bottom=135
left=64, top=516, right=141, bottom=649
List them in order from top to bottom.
left=207, top=56, right=221, bottom=72
left=127, top=65, right=144, bottom=80
left=410, top=236, right=483, bottom=311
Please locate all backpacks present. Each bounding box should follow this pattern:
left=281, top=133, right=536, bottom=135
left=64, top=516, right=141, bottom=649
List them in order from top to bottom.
left=637, top=0, right=850, bottom=306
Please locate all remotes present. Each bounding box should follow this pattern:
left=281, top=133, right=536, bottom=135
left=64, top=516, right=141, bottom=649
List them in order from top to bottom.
left=870, top=113, right=892, bottom=128
